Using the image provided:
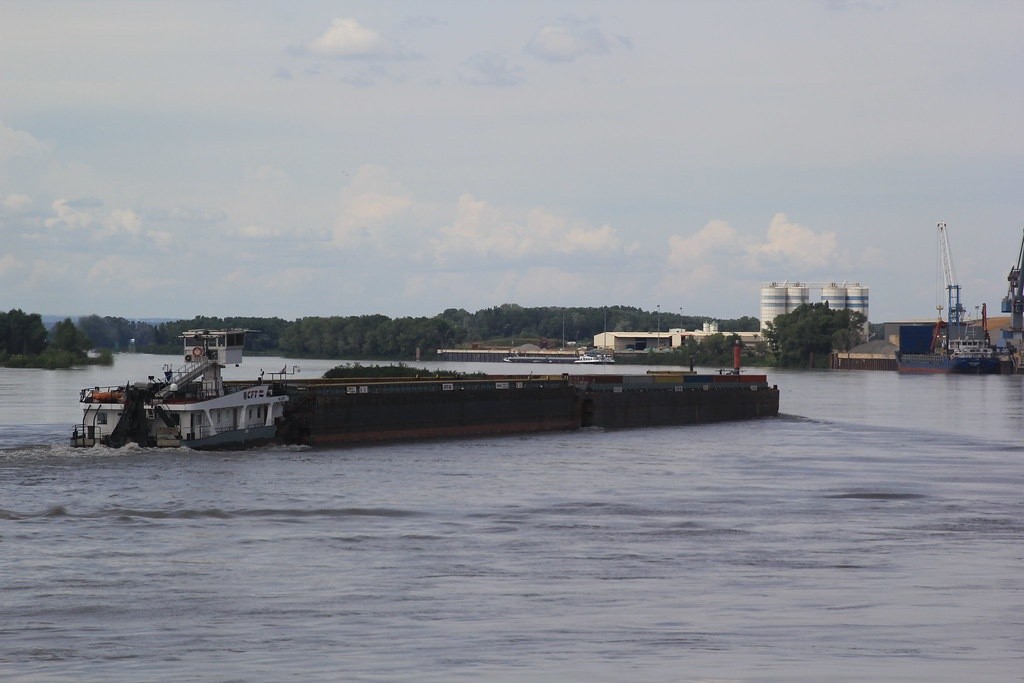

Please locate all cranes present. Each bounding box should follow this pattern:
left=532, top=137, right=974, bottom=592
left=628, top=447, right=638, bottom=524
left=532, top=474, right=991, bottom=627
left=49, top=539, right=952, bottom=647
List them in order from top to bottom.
left=896, top=220, right=1015, bottom=376
left=995, top=229, right=1024, bottom=374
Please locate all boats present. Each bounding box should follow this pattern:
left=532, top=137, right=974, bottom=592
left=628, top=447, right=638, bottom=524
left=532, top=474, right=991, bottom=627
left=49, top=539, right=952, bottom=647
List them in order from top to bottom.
left=70, top=328, right=780, bottom=449
left=574, top=354, right=615, bottom=363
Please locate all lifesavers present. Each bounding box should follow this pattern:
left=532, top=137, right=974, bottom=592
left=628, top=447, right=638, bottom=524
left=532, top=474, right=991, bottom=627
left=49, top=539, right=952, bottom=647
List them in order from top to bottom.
left=193, top=346, right=203, bottom=356
